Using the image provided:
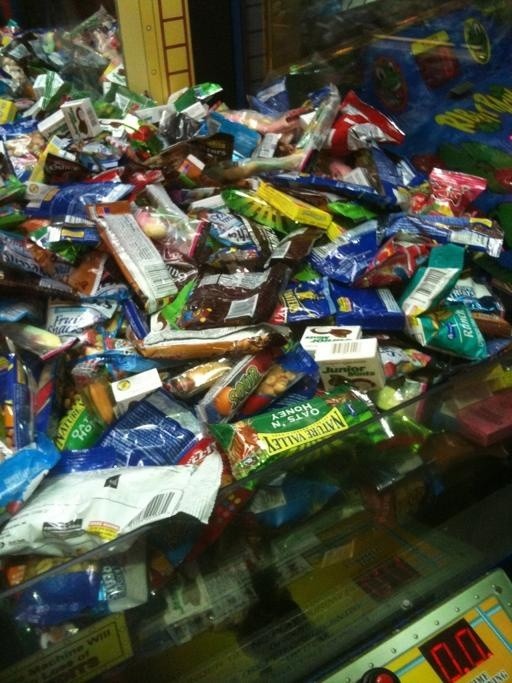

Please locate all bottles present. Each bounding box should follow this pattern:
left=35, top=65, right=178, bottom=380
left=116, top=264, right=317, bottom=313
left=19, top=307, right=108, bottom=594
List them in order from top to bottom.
left=285, top=64, right=304, bottom=107
